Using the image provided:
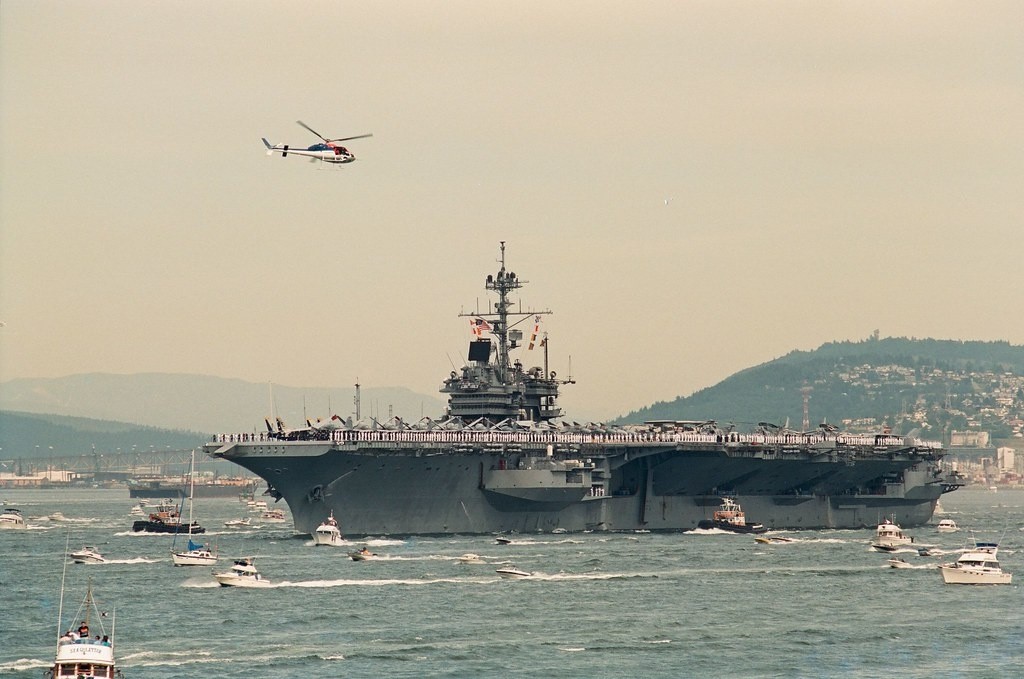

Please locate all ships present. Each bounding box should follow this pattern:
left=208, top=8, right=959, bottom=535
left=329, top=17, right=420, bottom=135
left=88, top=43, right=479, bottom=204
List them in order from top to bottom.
left=202, top=240, right=967, bottom=535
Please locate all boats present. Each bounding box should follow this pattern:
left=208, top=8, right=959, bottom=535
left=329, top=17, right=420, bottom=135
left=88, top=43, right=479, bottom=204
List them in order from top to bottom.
left=172, top=450, right=218, bottom=567
left=174, top=476, right=258, bottom=498
left=128, top=485, right=191, bottom=499
left=711, top=497, right=767, bottom=535
left=243, top=501, right=267, bottom=513
left=0, top=508, right=26, bottom=529
left=259, top=508, right=286, bottom=524
left=310, top=509, right=342, bottom=546
left=48, top=510, right=64, bottom=520
left=874, top=511, right=914, bottom=544
left=224, top=517, right=252, bottom=528
left=939, top=530, right=1013, bottom=585
left=49, top=534, right=123, bottom=679
left=495, top=537, right=510, bottom=543
left=871, top=542, right=898, bottom=552
left=69, top=545, right=105, bottom=565
left=346, top=548, right=378, bottom=561
left=919, top=549, right=933, bottom=556
left=216, top=550, right=270, bottom=587
left=460, top=552, right=481, bottom=562
left=887, top=557, right=915, bottom=569
left=496, top=562, right=531, bottom=577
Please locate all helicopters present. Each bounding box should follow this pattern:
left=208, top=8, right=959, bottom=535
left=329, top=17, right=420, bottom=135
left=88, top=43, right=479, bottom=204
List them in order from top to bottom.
left=260, top=121, right=375, bottom=171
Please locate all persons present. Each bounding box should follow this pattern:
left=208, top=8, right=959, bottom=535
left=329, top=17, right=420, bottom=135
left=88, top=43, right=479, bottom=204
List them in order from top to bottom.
left=211, top=431, right=901, bottom=447
left=59, top=620, right=112, bottom=649
